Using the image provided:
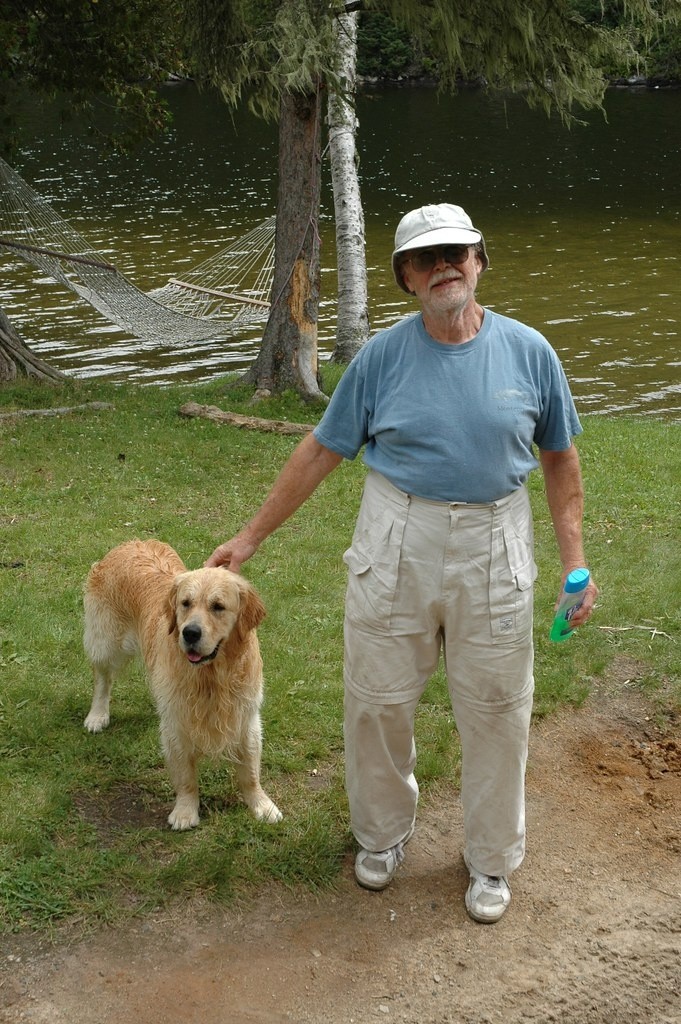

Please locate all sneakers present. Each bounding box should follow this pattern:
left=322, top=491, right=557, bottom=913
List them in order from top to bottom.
left=355, top=845, right=397, bottom=891
left=464, top=850, right=512, bottom=924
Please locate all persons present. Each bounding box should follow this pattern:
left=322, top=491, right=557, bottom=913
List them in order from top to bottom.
left=201, top=203, right=600, bottom=923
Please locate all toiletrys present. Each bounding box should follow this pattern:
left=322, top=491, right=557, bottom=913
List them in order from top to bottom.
left=549, top=567, right=590, bottom=644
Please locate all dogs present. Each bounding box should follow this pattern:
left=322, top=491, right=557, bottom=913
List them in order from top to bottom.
left=82, top=537, right=284, bottom=830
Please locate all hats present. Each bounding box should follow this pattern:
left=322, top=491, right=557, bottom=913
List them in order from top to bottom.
left=392, top=203, right=488, bottom=295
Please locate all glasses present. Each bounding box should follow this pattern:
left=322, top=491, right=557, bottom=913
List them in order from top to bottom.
left=400, top=244, right=472, bottom=272
